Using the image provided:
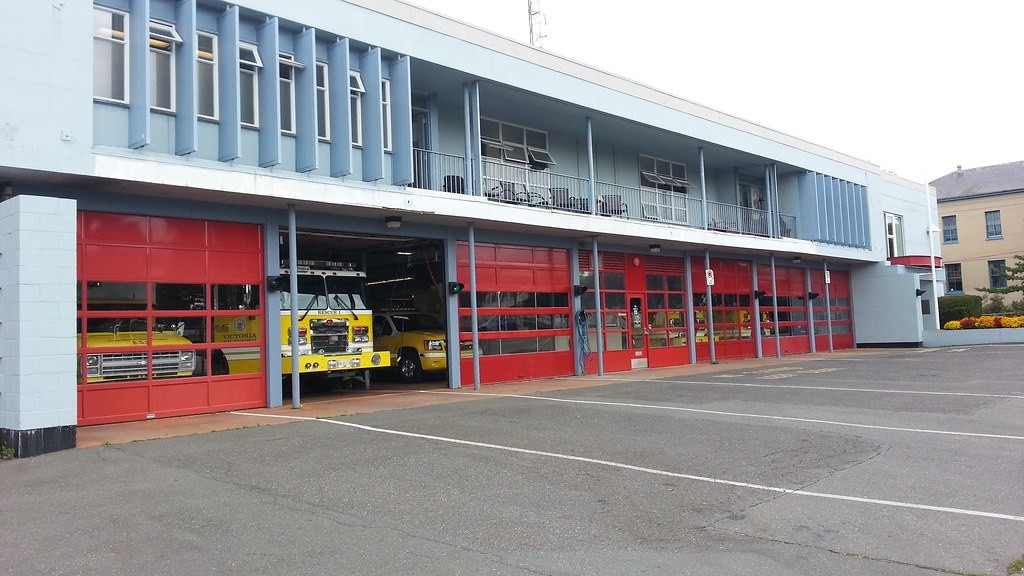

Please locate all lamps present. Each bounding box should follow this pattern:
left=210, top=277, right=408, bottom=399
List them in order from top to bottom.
left=384, top=216, right=402, bottom=228
left=648, top=244, right=661, bottom=253
left=792, top=256, right=801, bottom=264
left=267, top=275, right=283, bottom=292
left=448, top=282, right=464, bottom=295
left=574, top=285, right=588, bottom=296
left=754, top=291, right=766, bottom=298
left=808, top=292, right=819, bottom=300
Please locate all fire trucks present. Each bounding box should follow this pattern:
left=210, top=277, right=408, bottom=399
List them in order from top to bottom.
left=160, top=256, right=392, bottom=376
left=77, top=279, right=196, bottom=387
left=641, top=291, right=720, bottom=345
left=704, top=298, right=777, bottom=340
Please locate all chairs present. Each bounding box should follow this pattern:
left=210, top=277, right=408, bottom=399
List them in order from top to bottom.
left=443, top=175, right=464, bottom=194
left=486, top=181, right=506, bottom=201
left=504, top=181, right=531, bottom=208
left=523, top=184, right=548, bottom=209
left=549, top=188, right=575, bottom=211
left=600, top=195, right=629, bottom=219
left=712, top=217, right=737, bottom=233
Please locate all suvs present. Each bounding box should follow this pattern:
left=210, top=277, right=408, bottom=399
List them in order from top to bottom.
left=370, top=306, right=489, bottom=382
